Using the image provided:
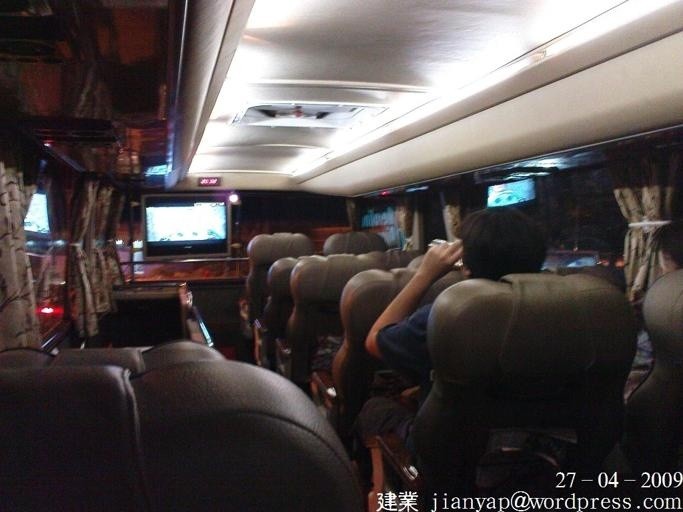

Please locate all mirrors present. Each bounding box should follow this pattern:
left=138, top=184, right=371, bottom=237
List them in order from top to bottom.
left=16, top=154, right=71, bottom=350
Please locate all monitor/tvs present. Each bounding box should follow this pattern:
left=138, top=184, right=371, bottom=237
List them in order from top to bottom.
left=485, top=177, right=537, bottom=211
left=23, top=190, right=52, bottom=240
left=141, top=192, right=230, bottom=261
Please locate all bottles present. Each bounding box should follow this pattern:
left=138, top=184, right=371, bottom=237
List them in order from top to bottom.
left=117, top=131, right=141, bottom=174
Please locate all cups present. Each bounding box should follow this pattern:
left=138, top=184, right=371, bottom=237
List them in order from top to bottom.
left=426, top=239, right=462, bottom=267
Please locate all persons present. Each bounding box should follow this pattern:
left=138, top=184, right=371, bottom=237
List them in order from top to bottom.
left=351, top=206, right=547, bottom=512
left=622, top=220, right=683, bottom=404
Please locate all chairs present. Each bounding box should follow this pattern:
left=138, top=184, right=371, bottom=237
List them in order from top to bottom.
left=241, top=228, right=683, bottom=510
left=2, top=341, right=371, bottom=512
